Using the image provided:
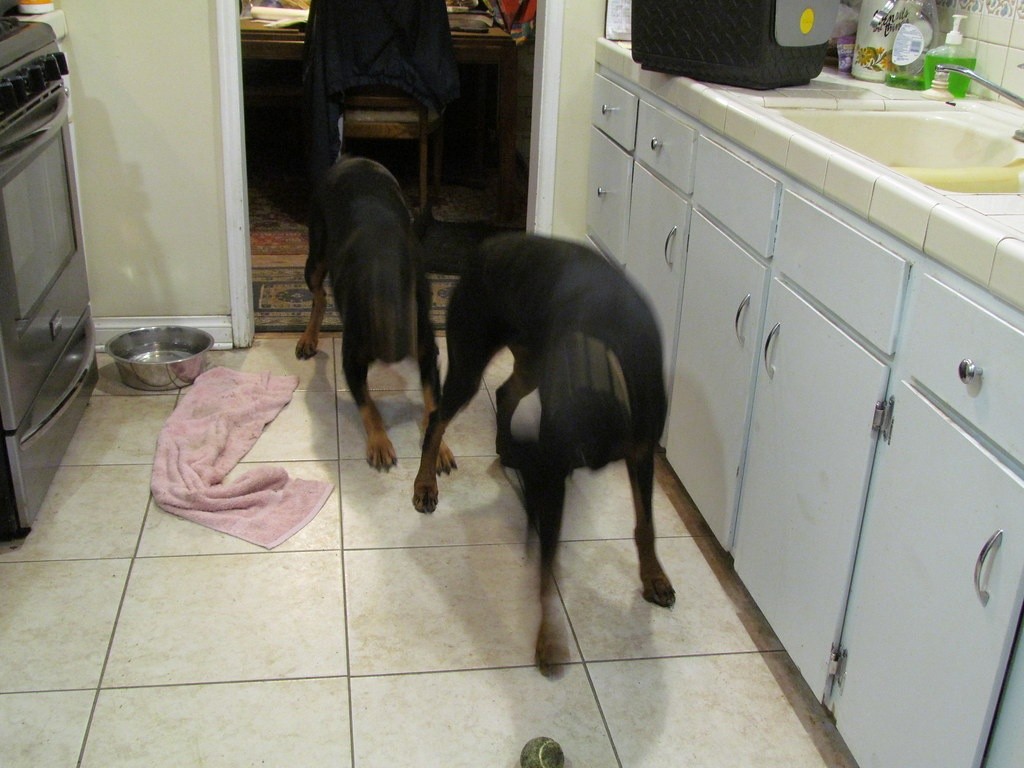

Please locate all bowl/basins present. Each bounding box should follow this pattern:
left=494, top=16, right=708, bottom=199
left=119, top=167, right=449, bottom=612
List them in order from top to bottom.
left=105, top=325, right=213, bottom=391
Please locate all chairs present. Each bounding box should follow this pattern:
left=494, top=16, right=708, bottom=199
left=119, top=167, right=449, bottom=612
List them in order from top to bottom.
left=314, top=1, right=448, bottom=217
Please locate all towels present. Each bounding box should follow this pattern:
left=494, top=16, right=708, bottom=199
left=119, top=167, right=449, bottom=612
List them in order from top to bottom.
left=150, top=365, right=335, bottom=551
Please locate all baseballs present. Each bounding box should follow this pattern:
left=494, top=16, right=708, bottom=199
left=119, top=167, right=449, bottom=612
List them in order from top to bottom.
left=520, top=737, right=564, bottom=768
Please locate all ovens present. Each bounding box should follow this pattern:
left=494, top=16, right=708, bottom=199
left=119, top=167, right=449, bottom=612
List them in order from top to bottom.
left=2, top=14, right=98, bottom=547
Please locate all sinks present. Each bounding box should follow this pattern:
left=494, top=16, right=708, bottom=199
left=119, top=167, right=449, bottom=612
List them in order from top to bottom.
left=777, top=106, right=1024, bottom=175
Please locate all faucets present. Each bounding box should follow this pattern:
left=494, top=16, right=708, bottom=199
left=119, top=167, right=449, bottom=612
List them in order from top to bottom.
left=935, top=63, right=1024, bottom=143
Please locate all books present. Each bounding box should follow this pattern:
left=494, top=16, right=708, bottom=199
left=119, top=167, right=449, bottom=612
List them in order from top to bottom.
left=445, top=10, right=493, bottom=33
left=276, top=19, right=308, bottom=29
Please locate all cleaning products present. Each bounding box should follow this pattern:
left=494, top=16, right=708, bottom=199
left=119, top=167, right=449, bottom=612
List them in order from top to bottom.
left=884, top=0, right=977, bottom=99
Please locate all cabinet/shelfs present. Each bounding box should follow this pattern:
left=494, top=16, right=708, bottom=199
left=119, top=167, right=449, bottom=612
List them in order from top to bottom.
left=581, top=71, right=639, bottom=273
left=624, top=99, right=698, bottom=451
left=663, top=132, right=915, bottom=710
left=833, top=266, right=1024, bottom=768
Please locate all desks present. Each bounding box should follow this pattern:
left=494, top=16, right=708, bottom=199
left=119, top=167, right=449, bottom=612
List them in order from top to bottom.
left=240, top=7, right=517, bottom=226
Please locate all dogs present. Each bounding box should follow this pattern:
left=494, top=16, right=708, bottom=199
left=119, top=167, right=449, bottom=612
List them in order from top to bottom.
left=295, top=156, right=459, bottom=478
left=414, top=231, right=679, bottom=682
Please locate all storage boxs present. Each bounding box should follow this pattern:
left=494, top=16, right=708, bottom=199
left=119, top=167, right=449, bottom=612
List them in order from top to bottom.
left=631, top=1, right=840, bottom=90
